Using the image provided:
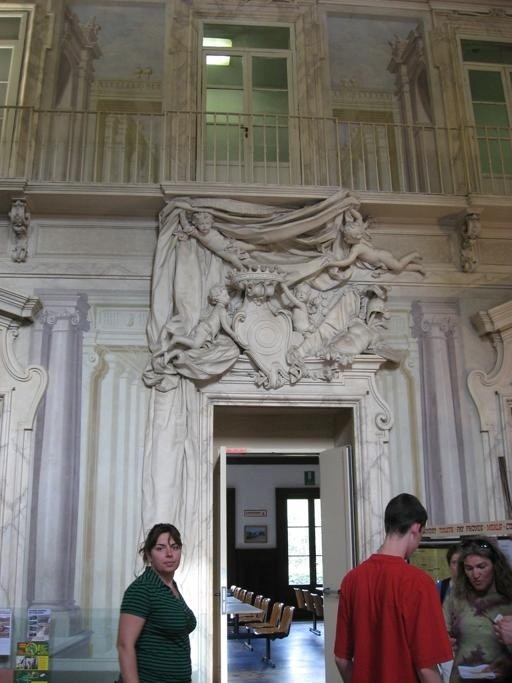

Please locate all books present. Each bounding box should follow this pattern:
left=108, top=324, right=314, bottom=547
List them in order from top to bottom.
left=28, top=607, right=51, bottom=641
left=15, top=641, right=50, bottom=683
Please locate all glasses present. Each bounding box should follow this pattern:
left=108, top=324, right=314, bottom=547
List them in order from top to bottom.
left=457, top=539, right=498, bottom=553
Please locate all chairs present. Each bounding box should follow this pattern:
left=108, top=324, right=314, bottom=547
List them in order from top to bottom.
left=226, top=585, right=323, bottom=667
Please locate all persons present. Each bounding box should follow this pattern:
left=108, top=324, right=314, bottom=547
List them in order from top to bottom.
left=335, top=493, right=454, bottom=683
left=117, top=523, right=196, bottom=683
left=441, top=534, right=512, bottom=683
left=437, top=544, right=458, bottom=607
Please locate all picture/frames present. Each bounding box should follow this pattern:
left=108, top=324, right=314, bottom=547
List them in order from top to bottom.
left=244, top=525, right=268, bottom=543
